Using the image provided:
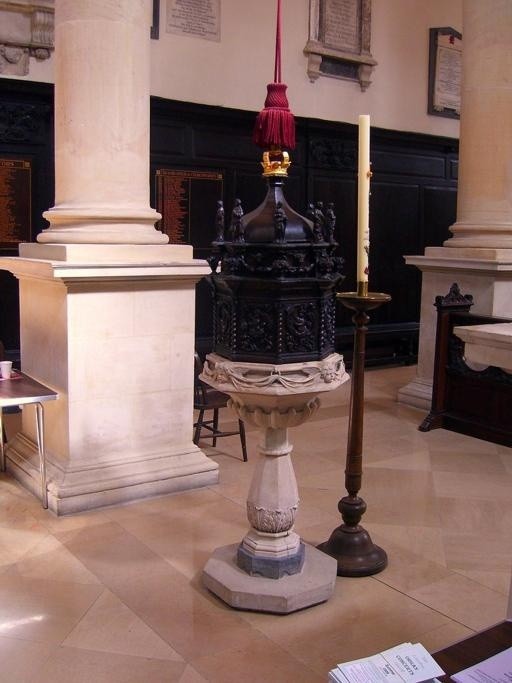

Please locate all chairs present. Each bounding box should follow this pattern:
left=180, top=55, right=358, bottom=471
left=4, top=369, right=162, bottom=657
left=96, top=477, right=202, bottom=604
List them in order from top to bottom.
left=193, top=351, right=248, bottom=462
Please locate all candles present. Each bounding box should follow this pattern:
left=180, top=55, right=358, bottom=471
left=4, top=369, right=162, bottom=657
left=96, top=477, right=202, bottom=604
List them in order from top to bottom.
left=357, top=114, right=372, bottom=281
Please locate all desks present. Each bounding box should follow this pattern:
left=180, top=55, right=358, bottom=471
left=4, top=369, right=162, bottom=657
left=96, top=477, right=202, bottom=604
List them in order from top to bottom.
left=0, top=368, right=59, bottom=509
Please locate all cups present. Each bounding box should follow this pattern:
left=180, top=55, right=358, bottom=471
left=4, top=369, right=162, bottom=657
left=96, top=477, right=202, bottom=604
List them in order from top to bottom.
left=0, top=361, right=12, bottom=379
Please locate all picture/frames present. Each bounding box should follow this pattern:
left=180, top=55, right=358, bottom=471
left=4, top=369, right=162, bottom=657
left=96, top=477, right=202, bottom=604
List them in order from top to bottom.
left=303, top=0, right=378, bottom=93
left=428, top=26, right=462, bottom=120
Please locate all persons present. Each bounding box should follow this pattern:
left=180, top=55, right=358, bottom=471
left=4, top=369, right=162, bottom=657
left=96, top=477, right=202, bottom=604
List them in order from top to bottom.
left=214, top=198, right=337, bottom=245
